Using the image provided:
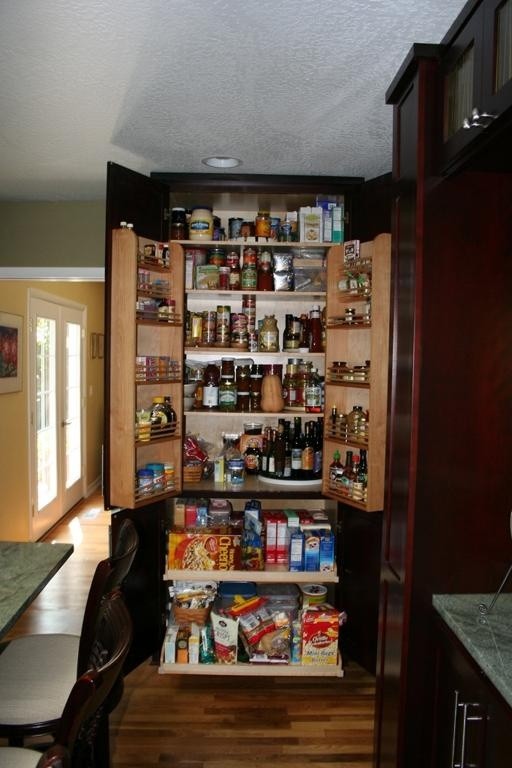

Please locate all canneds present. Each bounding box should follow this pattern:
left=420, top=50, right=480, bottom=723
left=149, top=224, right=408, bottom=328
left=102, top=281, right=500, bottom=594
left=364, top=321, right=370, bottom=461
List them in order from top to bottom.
left=172, top=206, right=186, bottom=222
left=227, top=211, right=297, bottom=243
left=172, top=223, right=185, bottom=240
left=208, top=247, right=239, bottom=267
left=217, top=358, right=263, bottom=412
left=283, top=358, right=311, bottom=407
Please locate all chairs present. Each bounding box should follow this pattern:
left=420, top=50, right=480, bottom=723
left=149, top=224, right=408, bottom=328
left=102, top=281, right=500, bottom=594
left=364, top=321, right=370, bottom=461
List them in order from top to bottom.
left=1, top=508, right=143, bottom=747
left=0, top=589, right=133, bottom=768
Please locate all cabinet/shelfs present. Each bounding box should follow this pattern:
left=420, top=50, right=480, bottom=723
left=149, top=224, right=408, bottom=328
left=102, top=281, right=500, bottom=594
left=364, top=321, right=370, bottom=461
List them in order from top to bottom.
left=374, top=0, right=510, bottom=768
left=427, top=592, right=512, bottom=767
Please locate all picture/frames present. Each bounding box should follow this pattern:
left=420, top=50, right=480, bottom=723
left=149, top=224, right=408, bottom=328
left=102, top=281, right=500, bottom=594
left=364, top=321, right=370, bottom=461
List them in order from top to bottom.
left=0, top=311, right=24, bottom=394
left=90, top=333, right=97, bottom=359
left=98, top=333, right=104, bottom=359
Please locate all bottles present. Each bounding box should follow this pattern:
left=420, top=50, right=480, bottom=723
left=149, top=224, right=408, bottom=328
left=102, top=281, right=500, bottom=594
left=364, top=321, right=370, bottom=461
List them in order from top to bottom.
left=171, top=205, right=292, bottom=242
left=138, top=271, right=150, bottom=289
left=157, top=297, right=175, bottom=323
left=283, top=304, right=327, bottom=353
left=258, top=314, right=279, bottom=351
left=183, top=354, right=324, bottom=413
left=330, top=360, right=369, bottom=381
left=151, top=396, right=175, bottom=438
left=329, top=404, right=368, bottom=442
left=223, top=415, right=323, bottom=485
left=329, top=449, right=367, bottom=504
left=138, top=463, right=175, bottom=498
left=301, top=584, right=327, bottom=607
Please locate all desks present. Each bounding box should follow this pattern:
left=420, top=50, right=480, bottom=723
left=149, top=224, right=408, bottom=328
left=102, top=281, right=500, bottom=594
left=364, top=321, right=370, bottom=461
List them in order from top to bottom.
left=0, top=541, right=74, bottom=639
